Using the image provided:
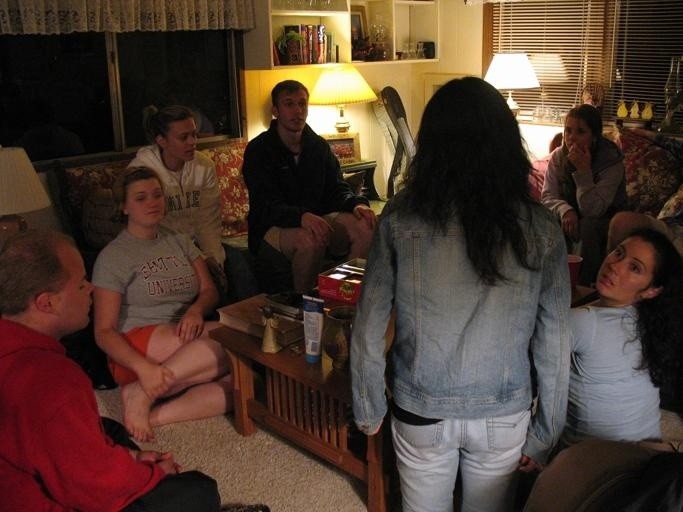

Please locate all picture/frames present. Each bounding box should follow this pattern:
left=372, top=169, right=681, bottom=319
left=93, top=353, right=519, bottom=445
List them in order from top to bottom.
left=318, top=132, right=361, bottom=165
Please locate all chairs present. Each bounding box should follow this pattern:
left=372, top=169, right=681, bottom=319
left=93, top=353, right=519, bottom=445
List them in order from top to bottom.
left=523, top=131, right=683, bottom=268
left=519, top=408, right=683, bottom=512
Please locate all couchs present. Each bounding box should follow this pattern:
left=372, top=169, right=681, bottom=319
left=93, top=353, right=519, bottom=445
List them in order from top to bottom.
left=58, top=139, right=348, bottom=300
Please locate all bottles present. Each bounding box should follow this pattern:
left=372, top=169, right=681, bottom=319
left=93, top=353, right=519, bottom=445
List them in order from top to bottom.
left=400, top=41, right=427, bottom=62
left=616, top=97, right=627, bottom=121
left=628, top=97, right=640, bottom=119
left=641, top=99, right=655, bottom=120
left=259, top=305, right=281, bottom=354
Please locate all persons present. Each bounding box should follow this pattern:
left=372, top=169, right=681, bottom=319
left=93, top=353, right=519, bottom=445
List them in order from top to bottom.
left=349, top=76, right=570, bottom=512
left=240, top=80, right=376, bottom=292
left=126, top=103, right=266, bottom=390
left=540, top=105, right=625, bottom=287
left=606, top=127, right=682, bottom=258
left=90, top=166, right=262, bottom=440
left=544, top=226, right=681, bottom=467
left=1, top=230, right=221, bottom=510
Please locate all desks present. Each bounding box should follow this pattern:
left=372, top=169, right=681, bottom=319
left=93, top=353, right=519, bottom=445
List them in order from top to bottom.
left=339, top=160, right=388, bottom=205
left=208, top=246, right=598, bottom=512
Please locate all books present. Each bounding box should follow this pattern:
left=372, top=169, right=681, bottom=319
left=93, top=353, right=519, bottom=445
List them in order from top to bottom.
left=270, top=22, right=340, bottom=67
left=265, top=288, right=304, bottom=320
left=216, top=293, right=330, bottom=348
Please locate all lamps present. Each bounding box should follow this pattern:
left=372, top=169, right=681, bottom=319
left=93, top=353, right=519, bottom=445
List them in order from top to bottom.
left=526, top=53, right=569, bottom=118
left=483, top=54, right=541, bottom=119
left=308, top=67, right=378, bottom=133
left=0, top=147, right=52, bottom=249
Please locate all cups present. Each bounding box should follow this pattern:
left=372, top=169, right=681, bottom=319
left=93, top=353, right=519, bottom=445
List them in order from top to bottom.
left=532, top=106, right=566, bottom=127
left=567, top=253, right=582, bottom=289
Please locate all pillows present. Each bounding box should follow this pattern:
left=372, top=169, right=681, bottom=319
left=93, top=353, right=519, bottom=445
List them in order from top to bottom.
left=612, top=128, right=683, bottom=221
left=80, top=189, right=127, bottom=246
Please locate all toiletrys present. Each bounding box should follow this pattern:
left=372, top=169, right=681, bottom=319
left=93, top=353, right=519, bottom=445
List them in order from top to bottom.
left=301, top=294, right=324, bottom=363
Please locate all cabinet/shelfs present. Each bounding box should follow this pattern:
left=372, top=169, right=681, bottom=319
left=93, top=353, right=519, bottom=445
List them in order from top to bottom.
left=244, top=0, right=440, bottom=70
